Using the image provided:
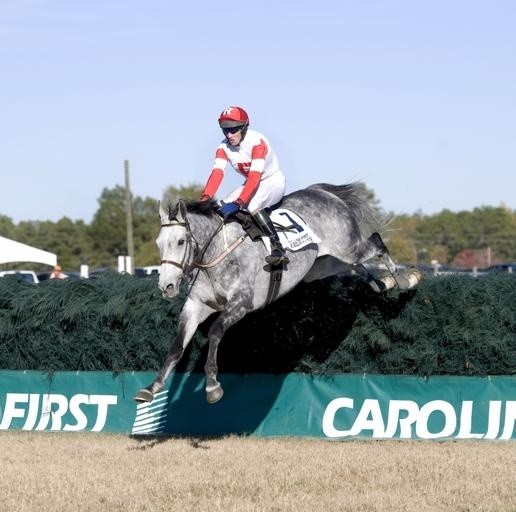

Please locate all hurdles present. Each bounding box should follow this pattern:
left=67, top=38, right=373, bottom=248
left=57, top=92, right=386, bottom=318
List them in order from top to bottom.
left=0, top=271, right=516, bottom=440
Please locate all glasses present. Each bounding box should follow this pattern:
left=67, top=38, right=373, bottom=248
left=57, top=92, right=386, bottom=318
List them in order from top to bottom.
left=223, top=128, right=239, bottom=133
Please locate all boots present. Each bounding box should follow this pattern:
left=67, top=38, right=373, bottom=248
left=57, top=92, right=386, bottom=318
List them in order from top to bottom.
left=252, top=209, right=289, bottom=266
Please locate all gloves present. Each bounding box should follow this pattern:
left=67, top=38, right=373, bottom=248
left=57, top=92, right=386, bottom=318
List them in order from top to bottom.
left=218, top=202, right=239, bottom=216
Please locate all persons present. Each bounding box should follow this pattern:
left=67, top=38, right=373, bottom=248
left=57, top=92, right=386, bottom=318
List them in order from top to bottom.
left=198, top=106, right=289, bottom=266
left=51, top=265, right=69, bottom=279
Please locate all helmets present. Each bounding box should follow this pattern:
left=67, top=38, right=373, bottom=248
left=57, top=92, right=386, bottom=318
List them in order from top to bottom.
left=218, top=107, right=249, bottom=128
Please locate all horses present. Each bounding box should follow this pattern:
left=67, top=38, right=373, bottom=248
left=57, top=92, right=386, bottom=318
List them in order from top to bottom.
left=132, top=180, right=423, bottom=405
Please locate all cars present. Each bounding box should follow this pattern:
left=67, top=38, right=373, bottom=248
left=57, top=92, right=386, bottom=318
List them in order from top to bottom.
left=0, top=266, right=160, bottom=284
